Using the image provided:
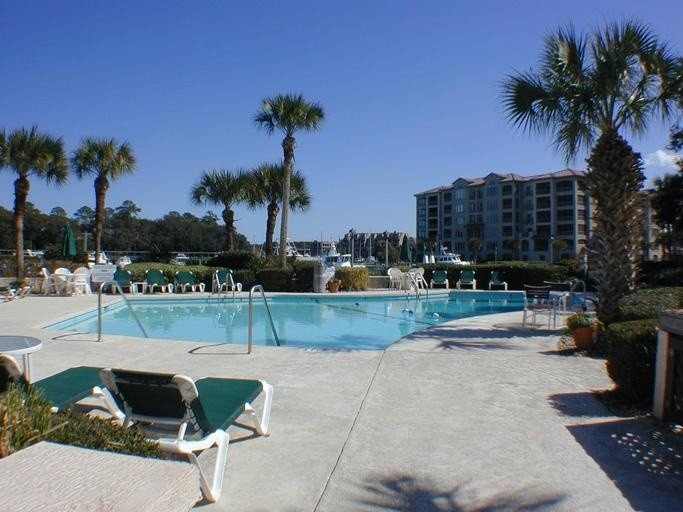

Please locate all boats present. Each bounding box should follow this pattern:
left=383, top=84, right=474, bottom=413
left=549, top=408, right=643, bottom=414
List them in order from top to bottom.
left=435, top=243, right=470, bottom=265
left=12, top=249, right=132, bottom=271
left=170, top=252, right=189, bottom=266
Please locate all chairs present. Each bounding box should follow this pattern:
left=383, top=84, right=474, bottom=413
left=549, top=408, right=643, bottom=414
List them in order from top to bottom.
left=44, top=264, right=243, bottom=296
left=387, top=267, right=508, bottom=292
left=523, top=282, right=579, bottom=332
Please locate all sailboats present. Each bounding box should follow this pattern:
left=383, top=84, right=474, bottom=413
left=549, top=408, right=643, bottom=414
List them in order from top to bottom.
left=273, top=228, right=380, bottom=266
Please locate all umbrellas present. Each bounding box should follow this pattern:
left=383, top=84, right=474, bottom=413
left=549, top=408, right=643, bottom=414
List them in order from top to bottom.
left=61, top=220, right=76, bottom=256
left=398, top=233, right=411, bottom=267
left=398, top=314, right=410, bottom=338
